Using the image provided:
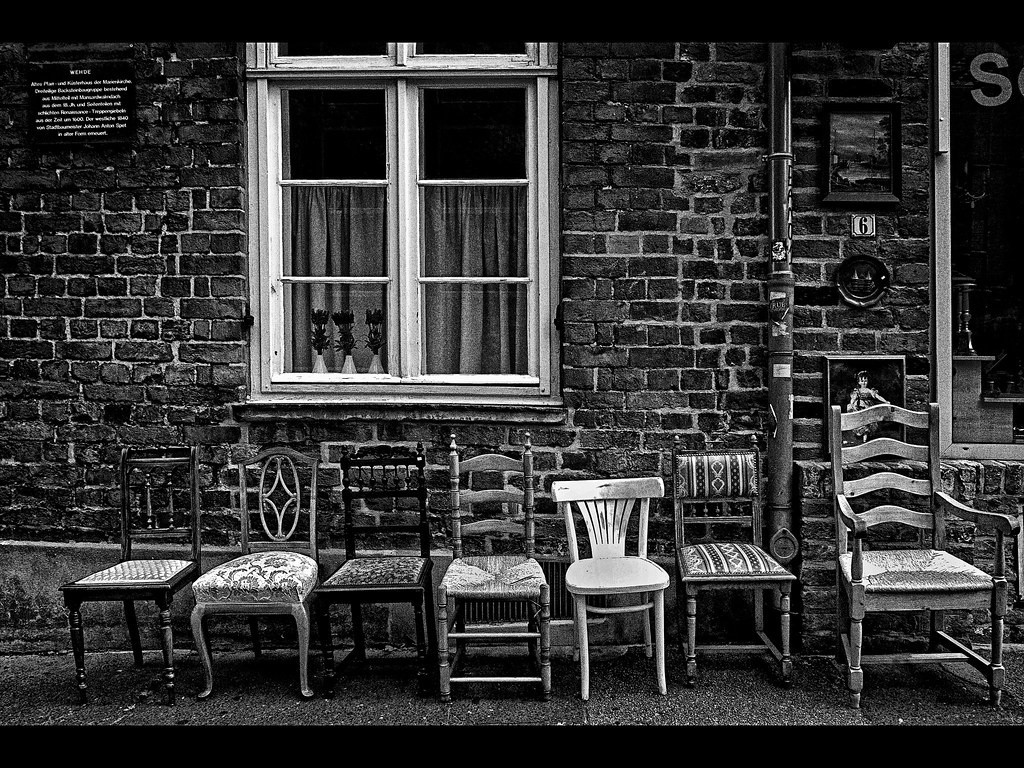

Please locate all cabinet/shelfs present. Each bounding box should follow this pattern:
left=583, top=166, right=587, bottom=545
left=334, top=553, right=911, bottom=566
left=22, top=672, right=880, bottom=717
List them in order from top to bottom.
left=951, top=354, right=1023, bottom=443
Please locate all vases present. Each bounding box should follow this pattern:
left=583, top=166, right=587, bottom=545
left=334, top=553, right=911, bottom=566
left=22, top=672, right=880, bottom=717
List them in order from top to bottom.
left=311, top=354, right=327, bottom=373
left=341, top=355, right=357, bottom=374
left=367, top=355, right=384, bottom=374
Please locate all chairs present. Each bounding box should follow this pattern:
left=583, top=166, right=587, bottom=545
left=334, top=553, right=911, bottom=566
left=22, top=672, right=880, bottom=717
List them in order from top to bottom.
left=830, top=401, right=1021, bottom=707
left=439, top=433, right=552, bottom=702
left=671, top=434, right=797, bottom=690
left=313, top=439, right=437, bottom=695
left=58, top=443, right=202, bottom=706
left=190, top=448, right=319, bottom=698
left=552, top=476, right=670, bottom=699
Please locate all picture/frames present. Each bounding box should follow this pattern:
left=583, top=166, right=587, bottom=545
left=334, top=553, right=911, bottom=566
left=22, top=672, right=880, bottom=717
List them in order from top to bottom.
left=818, top=101, right=903, bottom=211
left=823, top=353, right=906, bottom=462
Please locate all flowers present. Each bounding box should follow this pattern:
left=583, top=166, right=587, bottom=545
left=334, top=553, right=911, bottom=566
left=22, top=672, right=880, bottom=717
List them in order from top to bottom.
left=309, top=308, right=330, bottom=355
left=363, top=308, right=386, bottom=355
left=330, top=310, right=361, bottom=356
